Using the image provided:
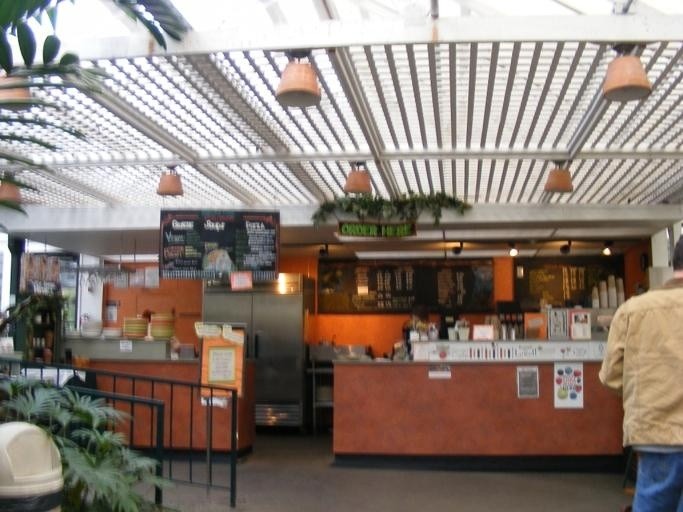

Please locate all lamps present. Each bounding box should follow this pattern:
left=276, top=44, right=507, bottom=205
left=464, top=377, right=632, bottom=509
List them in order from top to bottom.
left=600, top=42, right=652, bottom=101
left=274, top=50, right=320, bottom=108
left=342, top=161, right=372, bottom=195
left=543, top=161, right=574, bottom=194
left=157, top=166, right=185, bottom=195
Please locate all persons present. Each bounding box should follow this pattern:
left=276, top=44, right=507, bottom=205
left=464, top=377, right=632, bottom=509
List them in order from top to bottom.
left=598, top=233, right=683, bottom=511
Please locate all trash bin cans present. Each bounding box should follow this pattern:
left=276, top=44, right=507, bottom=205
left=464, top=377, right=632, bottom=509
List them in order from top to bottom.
left=0, top=420, right=68, bottom=512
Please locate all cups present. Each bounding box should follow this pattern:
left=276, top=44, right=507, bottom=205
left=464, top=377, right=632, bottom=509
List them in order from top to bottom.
left=592, top=277, right=624, bottom=308
left=499, top=325, right=516, bottom=340
left=449, top=326, right=470, bottom=340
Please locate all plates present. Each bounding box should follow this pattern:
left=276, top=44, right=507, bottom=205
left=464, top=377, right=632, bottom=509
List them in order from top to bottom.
left=66, top=314, right=177, bottom=339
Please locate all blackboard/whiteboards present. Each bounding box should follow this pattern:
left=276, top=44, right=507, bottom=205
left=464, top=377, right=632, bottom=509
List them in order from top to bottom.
left=158, top=207, right=282, bottom=287
left=512, top=253, right=629, bottom=313
left=314, top=255, right=497, bottom=315
